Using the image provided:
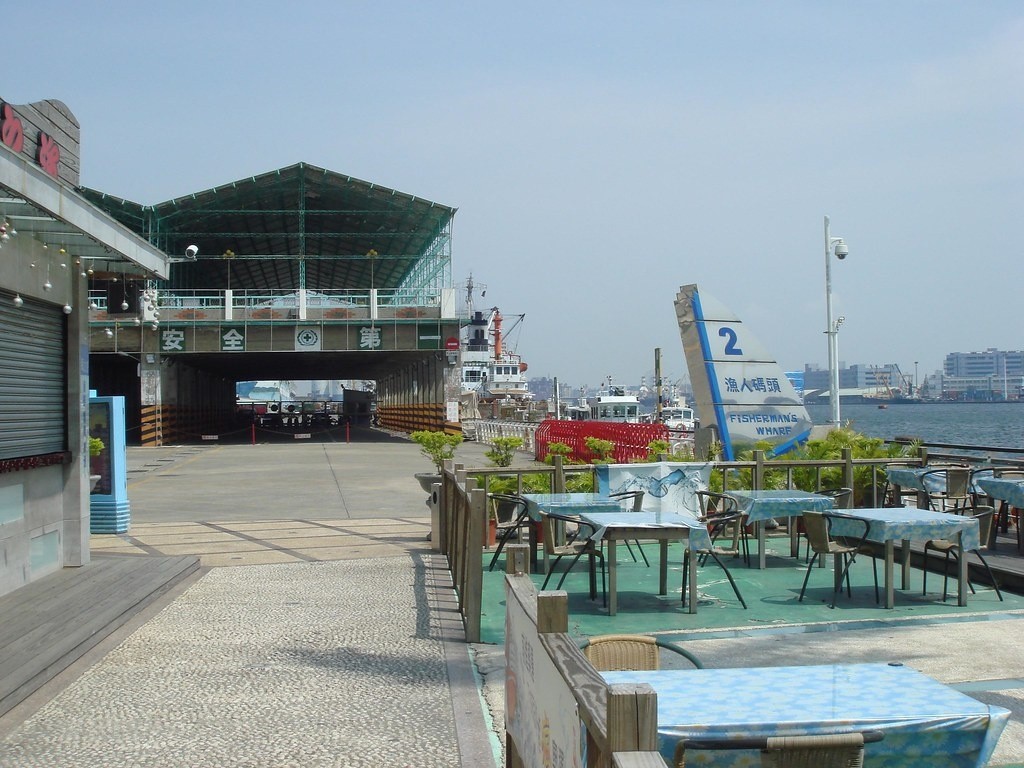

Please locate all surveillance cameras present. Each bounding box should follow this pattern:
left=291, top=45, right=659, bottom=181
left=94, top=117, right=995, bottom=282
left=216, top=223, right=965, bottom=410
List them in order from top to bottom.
left=185, top=245, right=199, bottom=259
left=835, top=245, right=848, bottom=259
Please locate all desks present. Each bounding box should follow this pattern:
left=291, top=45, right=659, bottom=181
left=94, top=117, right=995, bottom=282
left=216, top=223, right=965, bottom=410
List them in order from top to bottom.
left=885, top=469, right=995, bottom=513
left=976, top=477, right=1024, bottom=556
left=723, top=490, right=833, bottom=569
left=520, top=493, right=621, bottom=575
left=824, top=508, right=980, bottom=609
left=579, top=511, right=714, bottom=616
left=580, top=661, right=1012, bottom=768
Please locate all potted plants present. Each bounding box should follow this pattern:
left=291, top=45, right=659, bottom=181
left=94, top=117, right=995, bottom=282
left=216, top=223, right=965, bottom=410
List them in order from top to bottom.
left=402, top=430, right=465, bottom=495
left=482, top=434, right=525, bottom=524
left=89, top=436, right=105, bottom=493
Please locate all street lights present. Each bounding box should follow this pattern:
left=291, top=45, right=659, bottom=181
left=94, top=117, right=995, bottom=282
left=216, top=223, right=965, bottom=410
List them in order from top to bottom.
left=822, top=214, right=849, bottom=435
left=915, top=361, right=919, bottom=387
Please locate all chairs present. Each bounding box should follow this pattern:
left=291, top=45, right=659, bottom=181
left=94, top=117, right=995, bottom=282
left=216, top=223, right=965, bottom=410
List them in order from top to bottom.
left=881, top=463, right=1024, bottom=548
left=796, top=487, right=856, bottom=564
left=694, top=490, right=751, bottom=567
left=599, top=491, right=650, bottom=568
left=486, top=492, right=538, bottom=572
left=922, top=505, right=1003, bottom=602
left=681, top=509, right=747, bottom=610
left=538, top=510, right=606, bottom=608
left=798, top=510, right=880, bottom=609
left=577, top=634, right=704, bottom=671
left=674, top=730, right=886, bottom=768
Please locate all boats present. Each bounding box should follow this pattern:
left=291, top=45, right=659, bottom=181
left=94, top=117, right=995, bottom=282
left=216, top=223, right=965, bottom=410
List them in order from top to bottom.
left=455, top=271, right=697, bottom=433
left=878, top=403, right=888, bottom=409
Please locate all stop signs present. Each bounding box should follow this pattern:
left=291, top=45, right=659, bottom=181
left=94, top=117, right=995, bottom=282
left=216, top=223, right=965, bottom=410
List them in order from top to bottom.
left=446, top=338, right=458, bottom=351
